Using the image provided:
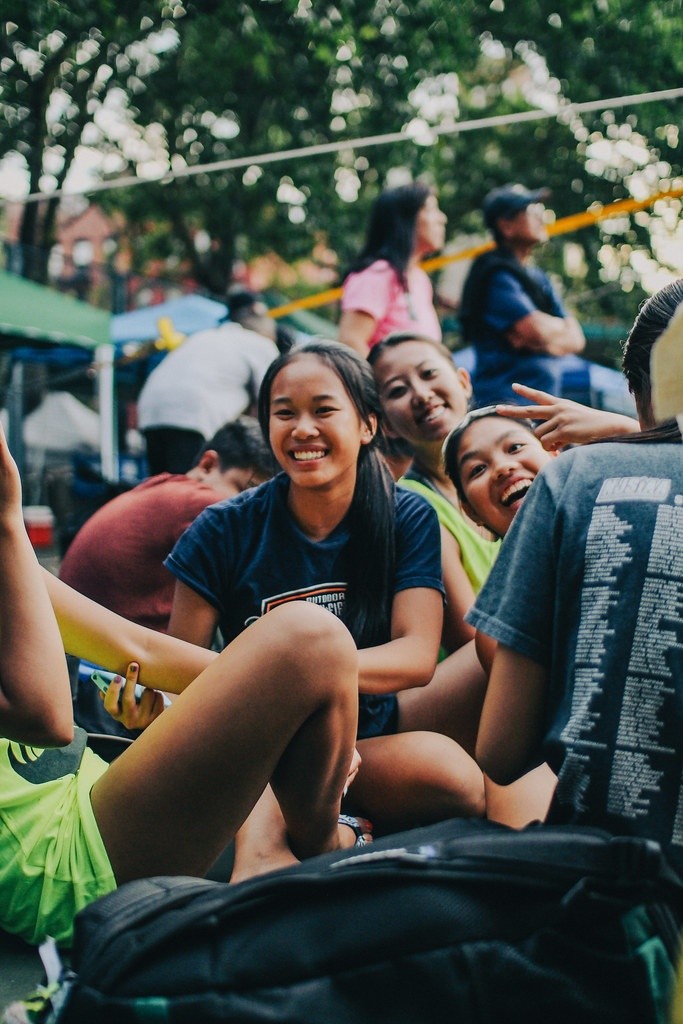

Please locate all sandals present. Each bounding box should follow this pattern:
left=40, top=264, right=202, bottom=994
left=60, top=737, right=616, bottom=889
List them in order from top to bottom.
left=338, top=815, right=366, bottom=849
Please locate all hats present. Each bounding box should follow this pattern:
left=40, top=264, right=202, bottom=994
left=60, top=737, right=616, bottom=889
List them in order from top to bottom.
left=484, top=182, right=551, bottom=222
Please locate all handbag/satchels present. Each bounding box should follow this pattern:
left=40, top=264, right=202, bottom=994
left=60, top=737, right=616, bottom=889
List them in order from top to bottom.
left=59, top=816, right=683, bottom=1024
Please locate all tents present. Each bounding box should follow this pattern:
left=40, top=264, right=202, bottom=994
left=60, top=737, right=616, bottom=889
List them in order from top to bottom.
left=0, top=265, right=120, bottom=482
left=111, top=294, right=229, bottom=340
left=451, top=345, right=627, bottom=410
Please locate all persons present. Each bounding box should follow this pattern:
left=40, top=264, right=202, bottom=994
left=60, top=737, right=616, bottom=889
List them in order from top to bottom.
left=338, top=183, right=586, bottom=411
left=0, top=294, right=683, bottom=943
